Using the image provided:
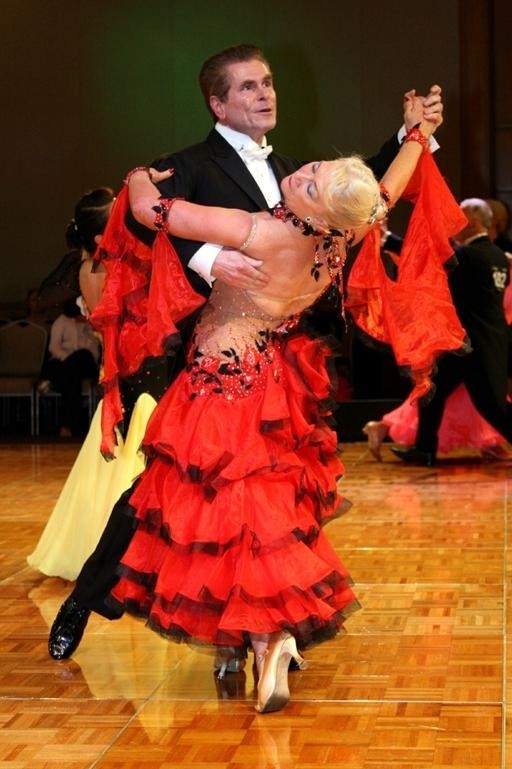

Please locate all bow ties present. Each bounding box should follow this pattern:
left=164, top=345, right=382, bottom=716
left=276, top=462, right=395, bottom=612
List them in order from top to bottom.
left=243, top=145, right=275, bottom=163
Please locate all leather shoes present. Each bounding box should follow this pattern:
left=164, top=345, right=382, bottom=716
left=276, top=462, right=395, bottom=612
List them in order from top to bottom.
left=392, top=447, right=438, bottom=468
left=47, top=593, right=92, bottom=661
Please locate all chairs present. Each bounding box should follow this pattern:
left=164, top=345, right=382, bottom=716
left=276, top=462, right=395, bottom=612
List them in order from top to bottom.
left=1, top=317, right=49, bottom=437
left=34, top=378, right=91, bottom=437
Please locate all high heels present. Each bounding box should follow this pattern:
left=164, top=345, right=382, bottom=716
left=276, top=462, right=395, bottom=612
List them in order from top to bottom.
left=363, top=420, right=385, bottom=462
left=253, top=627, right=307, bottom=713
left=214, top=645, right=248, bottom=680
